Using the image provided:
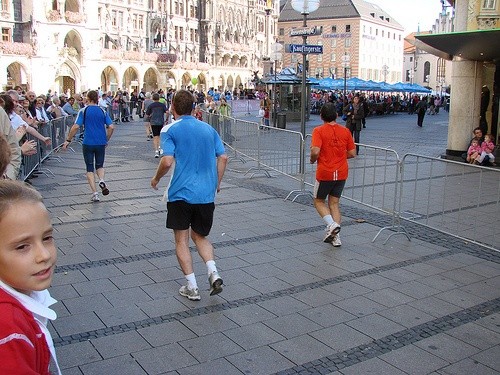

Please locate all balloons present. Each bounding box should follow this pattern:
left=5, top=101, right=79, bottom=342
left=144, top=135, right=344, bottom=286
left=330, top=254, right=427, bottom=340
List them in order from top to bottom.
left=191, top=78, right=198, bottom=85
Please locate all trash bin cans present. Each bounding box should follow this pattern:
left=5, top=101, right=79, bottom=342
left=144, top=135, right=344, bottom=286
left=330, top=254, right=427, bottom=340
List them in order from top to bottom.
left=277, top=114, right=286, bottom=128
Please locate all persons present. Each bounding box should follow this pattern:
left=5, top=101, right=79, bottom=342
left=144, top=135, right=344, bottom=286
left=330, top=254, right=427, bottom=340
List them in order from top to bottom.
left=481, top=85, right=490, bottom=136
left=0, top=86, right=496, bottom=181
left=151, top=90, right=228, bottom=300
left=63, top=91, right=114, bottom=202
left=310, top=103, right=355, bottom=247
left=0, top=180, right=62, bottom=375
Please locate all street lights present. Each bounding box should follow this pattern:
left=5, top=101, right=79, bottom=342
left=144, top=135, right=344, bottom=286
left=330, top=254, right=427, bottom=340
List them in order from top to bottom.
left=340, top=52, right=350, bottom=111
left=382, top=64, right=390, bottom=82
left=408, top=67, right=415, bottom=85
left=425, top=74, right=431, bottom=86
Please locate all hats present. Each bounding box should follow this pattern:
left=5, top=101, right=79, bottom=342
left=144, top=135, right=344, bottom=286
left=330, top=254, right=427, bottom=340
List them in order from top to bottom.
left=187, top=86, right=190, bottom=88
left=145, top=92, right=151, bottom=98
left=354, top=93, right=359, bottom=97
left=206, top=95, right=212, bottom=99
left=19, top=96, right=25, bottom=100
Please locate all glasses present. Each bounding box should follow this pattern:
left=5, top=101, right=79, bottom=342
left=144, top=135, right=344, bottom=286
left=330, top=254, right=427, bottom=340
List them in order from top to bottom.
left=29, top=95, right=34, bottom=97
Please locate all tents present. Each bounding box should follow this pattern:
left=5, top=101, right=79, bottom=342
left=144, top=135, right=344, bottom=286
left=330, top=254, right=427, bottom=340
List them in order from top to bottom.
left=307, top=77, right=432, bottom=100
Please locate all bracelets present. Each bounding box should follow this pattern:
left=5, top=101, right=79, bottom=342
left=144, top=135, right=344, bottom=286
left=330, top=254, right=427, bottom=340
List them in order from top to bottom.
left=66, top=140, right=71, bottom=143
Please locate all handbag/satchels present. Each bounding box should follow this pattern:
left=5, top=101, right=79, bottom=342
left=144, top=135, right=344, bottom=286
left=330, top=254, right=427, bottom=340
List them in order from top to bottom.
left=341, top=103, right=352, bottom=121
left=259, top=106, right=265, bottom=116
left=415, top=108, right=420, bottom=113
left=264, top=111, right=269, bottom=118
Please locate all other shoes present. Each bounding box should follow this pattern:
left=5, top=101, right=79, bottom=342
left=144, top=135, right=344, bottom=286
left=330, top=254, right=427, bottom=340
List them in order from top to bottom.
left=121, top=117, right=130, bottom=122
left=115, top=121, right=120, bottom=124
left=363, top=124, right=366, bottom=128
left=150, top=134, right=153, bottom=138
left=147, top=138, right=151, bottom=141
left=155, top=150, right=159, bottom=158
left=469, top=158, right=480, bottom=166
left=489, top=162, right=496, bottom=165
left=27, top=173, right=38, bottom=179
left=24, top=178, right=32, bottom=185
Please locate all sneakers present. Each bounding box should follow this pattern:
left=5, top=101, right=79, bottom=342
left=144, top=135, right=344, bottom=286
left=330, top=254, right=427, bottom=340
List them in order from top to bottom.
left=99, top=180, right=109, bottom=195
left=90, top=194, right=100, bottom=201
left=323, top=221, right=341, bottom=243
left=331, top=233, right=341, bottom=246
left=208, top=271, right=224, bottom=296
left=179, top=282, right=201, bottom=300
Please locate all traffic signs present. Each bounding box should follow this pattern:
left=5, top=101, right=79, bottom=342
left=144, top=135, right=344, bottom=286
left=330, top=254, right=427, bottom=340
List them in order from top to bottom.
left=289, top=25, right=323, bottom=37
left=284, top=43, right=324, bottom=53
